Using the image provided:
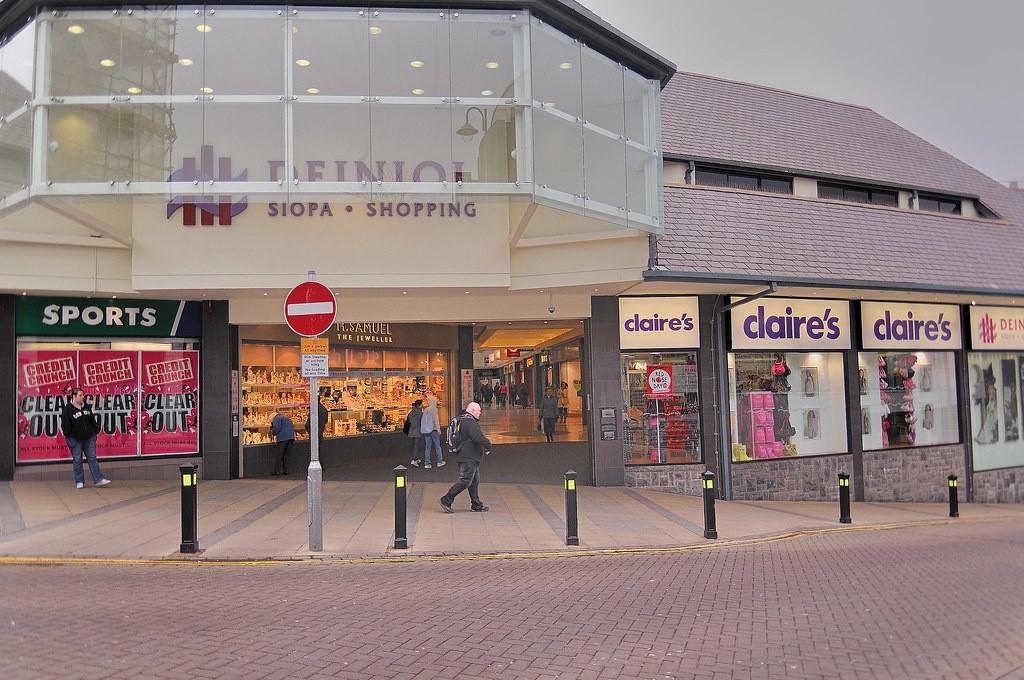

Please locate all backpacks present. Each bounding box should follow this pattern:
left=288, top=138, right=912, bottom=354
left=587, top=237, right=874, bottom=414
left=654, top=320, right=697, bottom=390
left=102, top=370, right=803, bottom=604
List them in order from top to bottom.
left=446, top=414, right=475, bottom=452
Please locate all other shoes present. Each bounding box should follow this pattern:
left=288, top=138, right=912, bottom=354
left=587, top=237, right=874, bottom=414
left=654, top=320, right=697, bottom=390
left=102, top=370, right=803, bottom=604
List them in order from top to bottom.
left=550, top=434, right=553, bottom=441
left=547, top=436, right=550, bottom=442
left=271, top=471, right=279, bottom=475
left=284, top=472, right=288, bottom=474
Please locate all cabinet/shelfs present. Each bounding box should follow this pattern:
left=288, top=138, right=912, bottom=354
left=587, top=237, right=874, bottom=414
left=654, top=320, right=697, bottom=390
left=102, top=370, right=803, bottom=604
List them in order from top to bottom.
left=735, top=351, right=790, bottom=458
left=622, top=365, right=700, bottom=466
left=243, top=380, right=448, bottom=446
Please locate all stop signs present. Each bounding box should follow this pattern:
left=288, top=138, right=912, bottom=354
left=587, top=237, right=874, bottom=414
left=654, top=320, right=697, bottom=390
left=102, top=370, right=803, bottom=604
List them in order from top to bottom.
left=283, top=281, right=337, bottom=337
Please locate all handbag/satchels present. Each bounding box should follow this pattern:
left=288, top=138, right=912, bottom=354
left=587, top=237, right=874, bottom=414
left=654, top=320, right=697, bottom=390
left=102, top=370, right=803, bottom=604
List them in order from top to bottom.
left=877, top=353, right=918, bottom=447
left=768, top=356, right=798, bottom=456
left=403, top=410, right=411, bottom=436
left=923, top=419, right=929, bottom=429
left=538, top=424, right=541, bottom=431
left=803, top=426, right=813, bottom=438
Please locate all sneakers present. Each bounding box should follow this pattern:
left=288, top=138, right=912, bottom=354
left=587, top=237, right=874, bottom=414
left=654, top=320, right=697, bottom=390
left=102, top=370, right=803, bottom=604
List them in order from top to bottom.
left=417, top=459, right=421, bottom=464
left=410, top=460, right=419, bottom=467
left=437, top=461, right=446, bottom=467
left=425, top=464, right=432, bottom=469
left=95, top=479, right=111, bottom=486
left=76, top=482, right=83, bottom=489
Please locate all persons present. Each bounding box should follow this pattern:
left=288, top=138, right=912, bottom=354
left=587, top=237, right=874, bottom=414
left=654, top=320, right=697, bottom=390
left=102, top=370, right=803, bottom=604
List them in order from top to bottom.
left=922, top=367, right=931, bottom=390
left=859, top=369, right=868, bottom=395
left=805, top=370, right=815, bottom=397
left=557, top=381, right=569, bottom=424
left=494, top=382, right=529, bottom=409
left=480, top=384, right=493, bottom=403
left=539, top=387, right=559, bottom=442
left=61, top=389, right=112, bottom=488
left=304, top=395, right=328, bottom=439
left=420, top=396, right=446, bottom=469
left=409, top=400, right=423, bottom=467
left=439, top=402, right=492, bottom=513
left=924, top=404, right=933, bottom=428
left=861, top=408, right=870, bottom=434
left=806, top=410, right=818, bottom=439
left=269, top=412, right=295, bottom=476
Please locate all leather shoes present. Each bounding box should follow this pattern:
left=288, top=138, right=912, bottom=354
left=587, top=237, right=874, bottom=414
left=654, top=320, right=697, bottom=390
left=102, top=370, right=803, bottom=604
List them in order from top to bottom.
left=439, top=498, right=455, bottom=512
left=470, top=505, right=489, bottom=512
